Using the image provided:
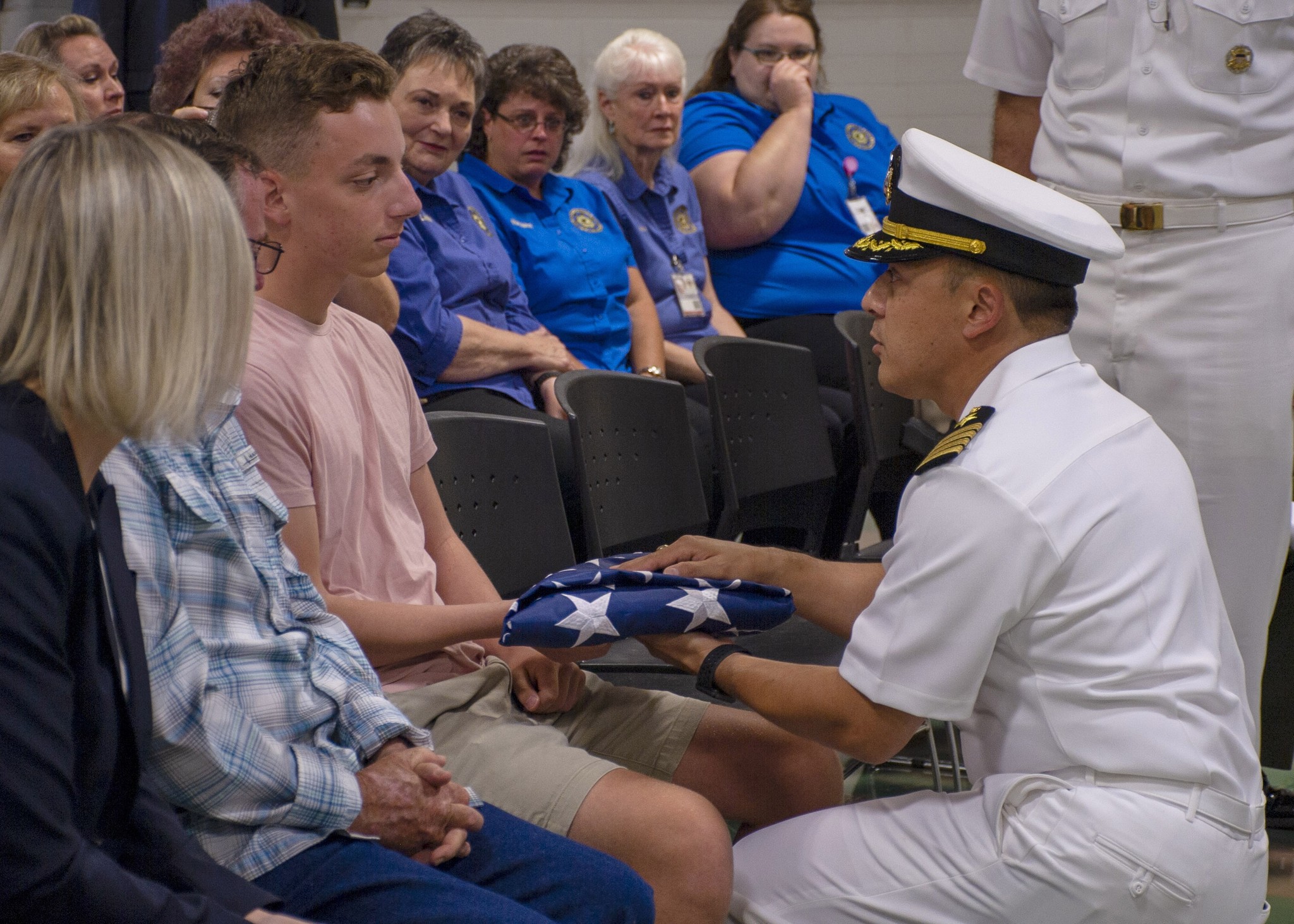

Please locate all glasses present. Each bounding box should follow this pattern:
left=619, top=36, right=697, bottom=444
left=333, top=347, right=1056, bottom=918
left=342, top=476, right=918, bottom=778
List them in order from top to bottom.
left=739, top=45, right=818, bottom=67
left=492, top=110, right=570, bottom=136
left=248, top=238, right=285, bottom=275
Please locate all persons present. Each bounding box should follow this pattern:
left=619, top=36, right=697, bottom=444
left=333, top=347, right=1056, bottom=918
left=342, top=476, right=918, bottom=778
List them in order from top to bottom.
left=964, top=1, right=1293, bottom=737
left=149, top=2, right=322, bottom=129
left=673, top=2, right=963, bottom=561
left=333, top=8, right=591, bottom=567
left=14, top=14, right=125, bottom=122
left=571, top=28, right=815, bottom=553
left=212, top=39, right=843, bottom=924
left=458, top=45, right=662, bottom=381
left=0, top=54, right=85, bottom=200
left=89, top=109, right=656, bottom=924
left=0, top=117, right=317, bottom=924
left=608, top=125, right=1269, bottom=922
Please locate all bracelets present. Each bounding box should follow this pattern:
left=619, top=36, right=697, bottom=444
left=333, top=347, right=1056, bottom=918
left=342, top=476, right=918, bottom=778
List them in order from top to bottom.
left=534, top=371, right=561, bottom=410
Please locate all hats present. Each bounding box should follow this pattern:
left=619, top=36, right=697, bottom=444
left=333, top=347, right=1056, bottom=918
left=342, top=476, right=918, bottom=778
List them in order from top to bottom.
left=844, top=127, right=1126, bottom=287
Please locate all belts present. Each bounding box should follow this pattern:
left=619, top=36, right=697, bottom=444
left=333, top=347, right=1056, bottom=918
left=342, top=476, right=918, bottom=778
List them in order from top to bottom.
left=1035, top=178, right=1294, bottom=230
left=1051, top=767, right=1267, bottom=835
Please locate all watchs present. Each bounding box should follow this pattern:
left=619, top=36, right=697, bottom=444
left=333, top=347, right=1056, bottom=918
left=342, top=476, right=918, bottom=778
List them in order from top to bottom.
left=635, top=366, right=665, bottom=379
left=695, top=644, right=751, bottom=704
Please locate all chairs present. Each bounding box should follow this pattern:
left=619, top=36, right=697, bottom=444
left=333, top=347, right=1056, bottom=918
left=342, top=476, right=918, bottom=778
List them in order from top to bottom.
left=427, top=304, right=963, bottom=799
